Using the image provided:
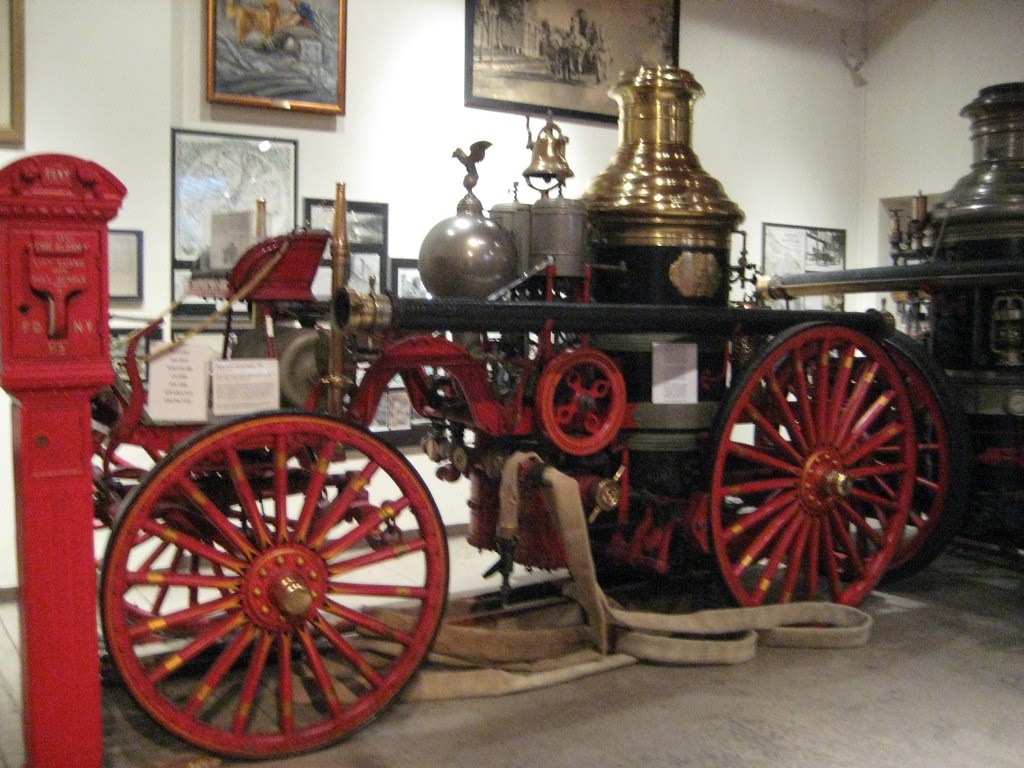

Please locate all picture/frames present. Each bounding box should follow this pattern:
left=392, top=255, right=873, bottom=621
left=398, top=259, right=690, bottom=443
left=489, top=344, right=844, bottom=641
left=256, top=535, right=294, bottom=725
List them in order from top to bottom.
left=0, top=0, right=26, bottom=146
left=207, top=0, right=347, bottom=116
left=465, top=0, right=681, bottom=123
